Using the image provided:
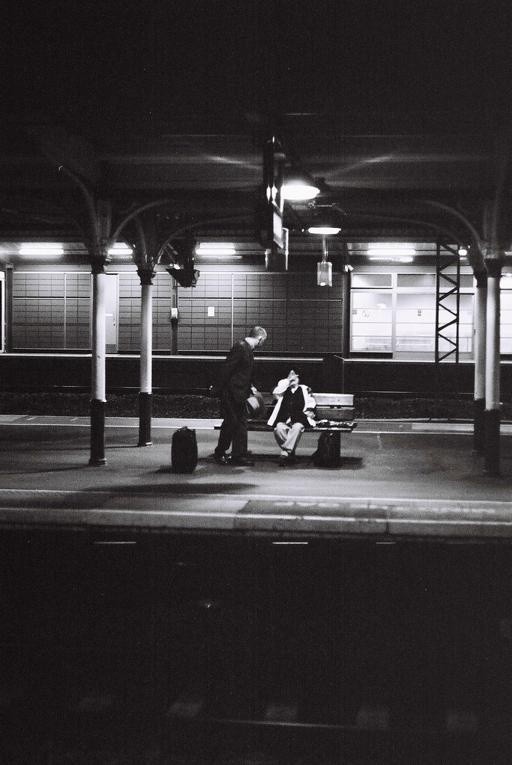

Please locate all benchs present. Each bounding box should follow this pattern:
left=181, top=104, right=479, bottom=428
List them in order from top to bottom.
left=214, top=390, right=356, bottom=468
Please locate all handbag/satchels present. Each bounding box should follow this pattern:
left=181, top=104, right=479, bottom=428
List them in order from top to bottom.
left=245, top=394, right=266, bottom=416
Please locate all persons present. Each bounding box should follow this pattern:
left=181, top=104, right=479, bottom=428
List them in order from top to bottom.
left=212, top=325, right=268, bottom=466
left=266, top=367, right=317, bottom=467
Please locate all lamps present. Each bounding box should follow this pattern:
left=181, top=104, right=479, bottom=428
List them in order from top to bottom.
left=280, top=166, right=342, bottom=237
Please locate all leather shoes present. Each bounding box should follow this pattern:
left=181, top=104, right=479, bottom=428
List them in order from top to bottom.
left=214, top=456, right=227, bottom=464
left=233, top=458, right=255, bottom=466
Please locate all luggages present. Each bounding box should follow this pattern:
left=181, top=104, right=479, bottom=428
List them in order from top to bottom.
left=171, top=426, right=197, bottom=474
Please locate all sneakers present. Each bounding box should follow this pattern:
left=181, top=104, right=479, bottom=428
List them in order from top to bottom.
left=280, top=450, right=288, bottom=460
